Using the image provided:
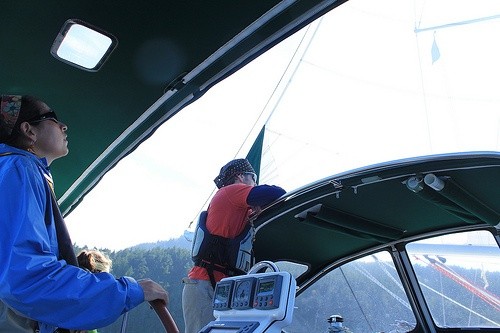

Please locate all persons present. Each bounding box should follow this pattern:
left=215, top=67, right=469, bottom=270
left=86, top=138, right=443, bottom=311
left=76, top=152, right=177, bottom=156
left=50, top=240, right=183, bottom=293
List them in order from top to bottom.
left=0, top=95, right=170, bottom=333
left=182, top=158, right=287, bottom=333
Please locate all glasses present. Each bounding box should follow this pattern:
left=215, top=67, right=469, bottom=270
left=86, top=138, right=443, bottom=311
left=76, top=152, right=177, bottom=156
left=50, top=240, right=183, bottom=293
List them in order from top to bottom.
left=30, top=110, right=58, bottom=124
left=235, top=172, right=257, bottom=183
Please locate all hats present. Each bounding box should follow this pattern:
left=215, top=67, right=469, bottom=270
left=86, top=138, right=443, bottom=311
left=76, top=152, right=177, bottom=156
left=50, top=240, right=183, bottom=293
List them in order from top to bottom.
left=0, top=95, right=22, bottom=140
left=214, top=159, right=255, bottom=188
